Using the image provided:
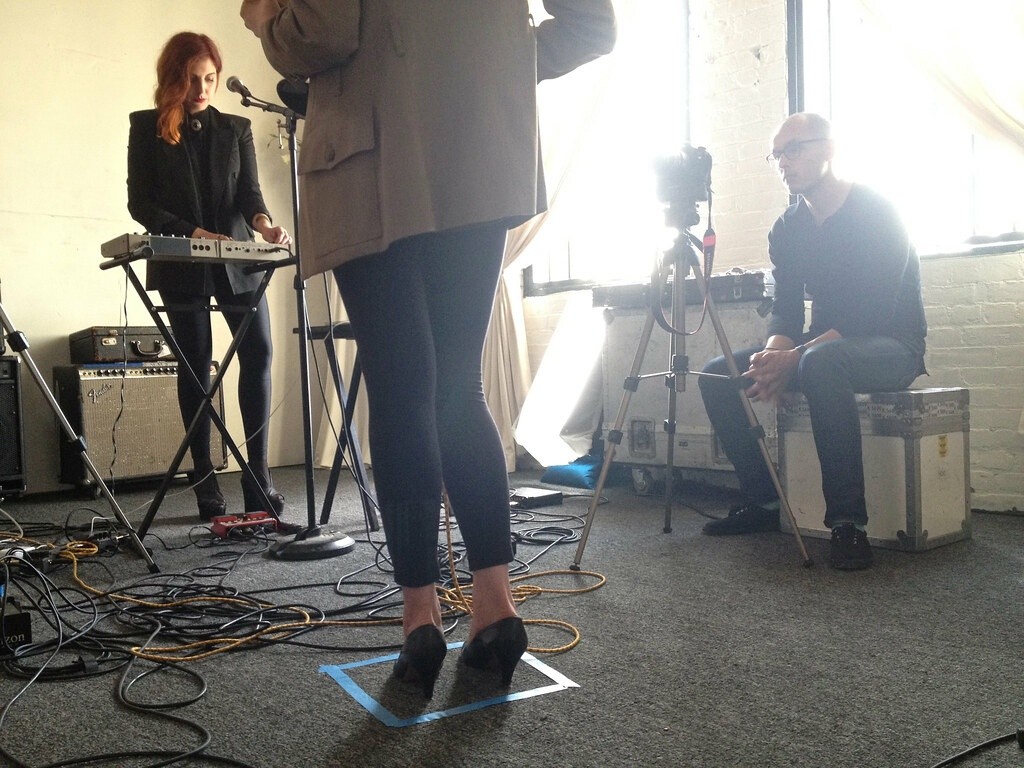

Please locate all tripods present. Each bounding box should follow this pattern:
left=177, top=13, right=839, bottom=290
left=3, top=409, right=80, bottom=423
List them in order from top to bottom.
left=569, top=204, right=815, bottom=573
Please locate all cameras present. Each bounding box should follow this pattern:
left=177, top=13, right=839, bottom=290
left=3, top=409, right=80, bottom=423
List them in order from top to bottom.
left=657, top=144, right=712, bottom=203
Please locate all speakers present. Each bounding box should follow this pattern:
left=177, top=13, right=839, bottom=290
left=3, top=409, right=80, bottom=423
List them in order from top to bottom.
left=0, top=355, right=26, bottom=498
left=52, top=362, right=229, bottom=483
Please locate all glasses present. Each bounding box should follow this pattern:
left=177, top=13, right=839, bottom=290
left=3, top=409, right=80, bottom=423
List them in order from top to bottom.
left=767, top=138, right=832, bottom=167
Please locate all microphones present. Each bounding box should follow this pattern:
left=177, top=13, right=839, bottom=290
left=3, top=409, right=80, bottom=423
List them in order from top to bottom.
left=226, top=76, right=251, bottom=96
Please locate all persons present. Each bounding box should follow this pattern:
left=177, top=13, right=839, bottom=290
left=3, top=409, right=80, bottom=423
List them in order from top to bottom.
left=241, top=0, right=620, bottom=709
left=125, top=29, right=294, bottom=522
left=694, top=107, right=933, bottom=572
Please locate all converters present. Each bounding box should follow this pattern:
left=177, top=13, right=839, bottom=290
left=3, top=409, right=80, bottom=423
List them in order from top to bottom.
left=20, top=550, right=56, bottom=573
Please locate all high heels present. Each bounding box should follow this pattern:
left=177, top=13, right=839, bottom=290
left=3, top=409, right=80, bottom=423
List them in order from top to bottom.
left=241, top=460, right=285, bottom=516
left=188, top=470, right=227, bottom=519
left=459, top=617, right=528, bottom=688
left=392, top=624, right=446, bottom=699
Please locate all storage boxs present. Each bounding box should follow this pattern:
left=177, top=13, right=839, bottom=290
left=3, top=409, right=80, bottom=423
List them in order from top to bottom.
left=0, top=356, right=27, bottom=501
left=53, top=361, right=228, bottom=500
left=777, top=388, right=970, bottom=553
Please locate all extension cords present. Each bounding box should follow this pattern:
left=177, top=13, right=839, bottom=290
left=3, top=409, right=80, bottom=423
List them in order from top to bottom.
left=210, top=510, right=279, bottom=540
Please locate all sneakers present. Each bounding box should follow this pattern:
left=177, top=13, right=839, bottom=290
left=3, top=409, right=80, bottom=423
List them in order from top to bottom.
left=702, top=504, right=780, bottom=536
left=830, top=522, right=873, bottom=569
